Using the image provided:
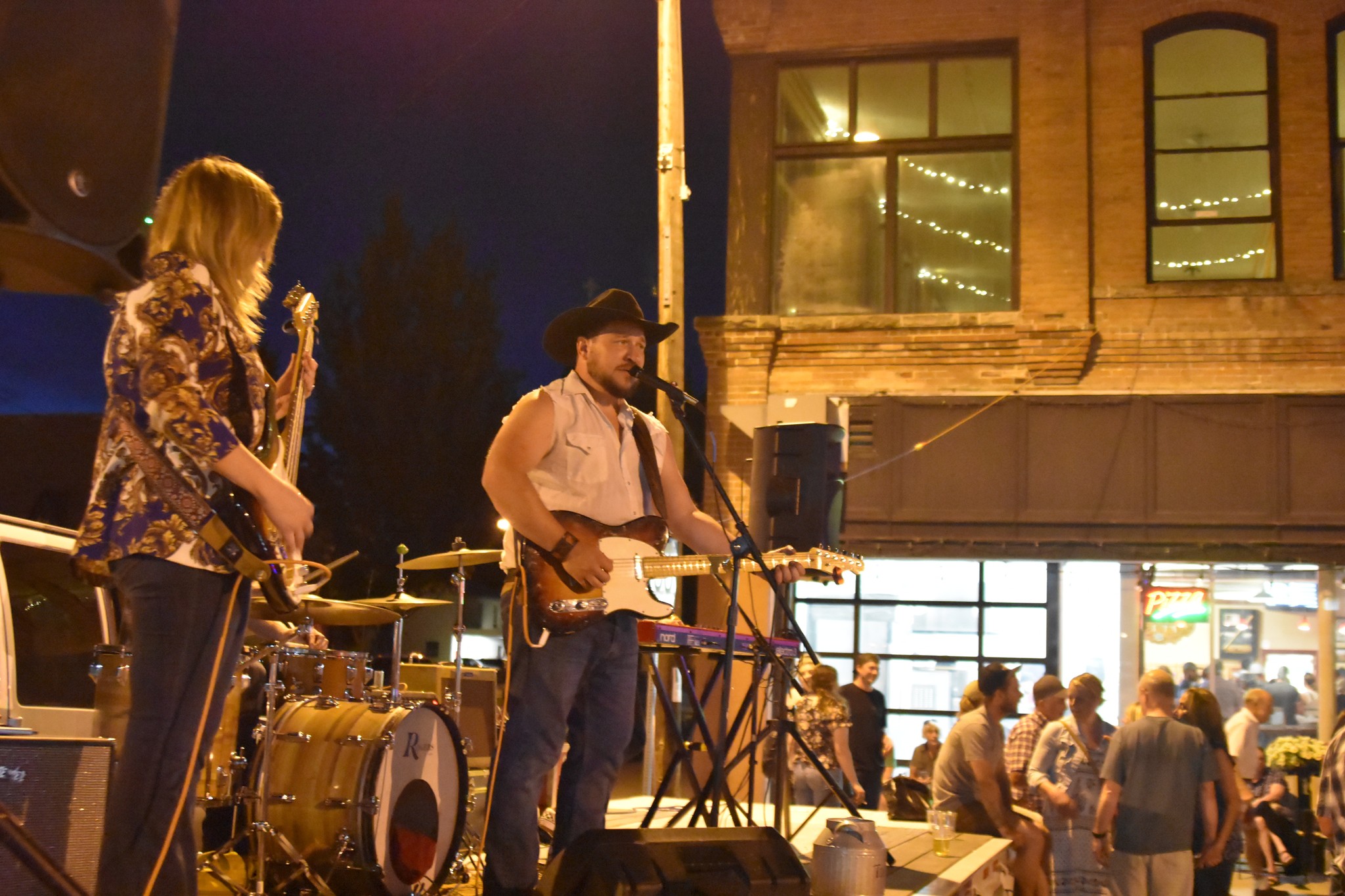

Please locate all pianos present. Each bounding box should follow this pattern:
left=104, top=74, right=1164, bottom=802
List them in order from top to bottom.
left=636, top=621, right=802, bottom=829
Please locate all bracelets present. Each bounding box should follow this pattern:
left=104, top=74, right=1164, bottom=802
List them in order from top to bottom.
left=550, top=530, right=579, bottom=564
left=1092, top=830, right=1109, bottom=838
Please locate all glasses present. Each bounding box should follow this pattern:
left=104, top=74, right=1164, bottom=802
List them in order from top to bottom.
left=924, top=719, right=937, bottom=724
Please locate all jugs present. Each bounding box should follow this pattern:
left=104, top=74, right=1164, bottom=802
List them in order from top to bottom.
left=811, top=816, right=887, bottom=896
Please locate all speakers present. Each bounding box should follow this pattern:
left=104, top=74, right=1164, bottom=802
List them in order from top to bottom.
left=0, top=0, right=180, bottom=298
left=750, top=423, right=845, bottom=581
left=0, top=513, right=119, bottom=738
left=400, top=663, right=497, bottom=768
left=0, top=733, right=117, bottom=896
left=526, top=826, right=812, bottom=896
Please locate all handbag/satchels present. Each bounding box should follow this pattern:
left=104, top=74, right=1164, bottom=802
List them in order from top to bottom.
left=881, top=775, right=932, bottom=822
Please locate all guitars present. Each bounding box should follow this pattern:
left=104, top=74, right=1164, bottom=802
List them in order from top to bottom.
left=236, top=282, right=322, bottom=618
left=520, top=521, right=865, bottom=631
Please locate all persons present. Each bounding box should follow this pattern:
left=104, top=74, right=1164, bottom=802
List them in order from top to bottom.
left=96, top=154, right=315, bottom=896
left=482, top=292, right=806, bottom=896
left=786, top=656, right=1345, bottom=896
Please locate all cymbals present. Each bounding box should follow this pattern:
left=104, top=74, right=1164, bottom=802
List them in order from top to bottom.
left=396, top=548, right=505, bottom=571
left=347, top=593, right=454, bottom=615
left=251, top=594, right=398, bottom=628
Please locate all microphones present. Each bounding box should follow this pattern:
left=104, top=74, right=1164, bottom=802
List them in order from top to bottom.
left=629, top=365, right=704, bottom=410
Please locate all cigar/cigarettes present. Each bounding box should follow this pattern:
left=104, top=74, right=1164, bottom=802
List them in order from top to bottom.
left=861, top=800, right=867, bottom=805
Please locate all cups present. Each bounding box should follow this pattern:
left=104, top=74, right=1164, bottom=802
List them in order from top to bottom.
left=926, top=809, right=957, bottom=856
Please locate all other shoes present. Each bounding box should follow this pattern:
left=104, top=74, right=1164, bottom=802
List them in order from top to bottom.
left=1278, top=850, right=1296, bottom=869
left=1266, top=871, right=1279, bottom=886
left=1254, top=887, right=1290, bottom=896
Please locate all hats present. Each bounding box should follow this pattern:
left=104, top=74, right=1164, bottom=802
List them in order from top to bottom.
left=542, top=289, right=681, bottom=369
left=798, top=652, right=821, bottom=666
left=978, top=662, right=1023, bottom=696
left=1184, top=662, right=1198, bottom=671
left=1033, top=675, right=1070, bottom=701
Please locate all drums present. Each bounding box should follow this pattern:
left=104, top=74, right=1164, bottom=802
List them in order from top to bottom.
left=267, top=645, right=370, bottom=702
left=93, top=646, right=243, bottom=810
left=242, top=692, right=471, bottom=896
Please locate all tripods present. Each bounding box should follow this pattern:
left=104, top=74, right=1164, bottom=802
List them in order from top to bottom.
left=666, top=583, right=895, bottom=866
left=195, top=599, right=336, bottom=895
